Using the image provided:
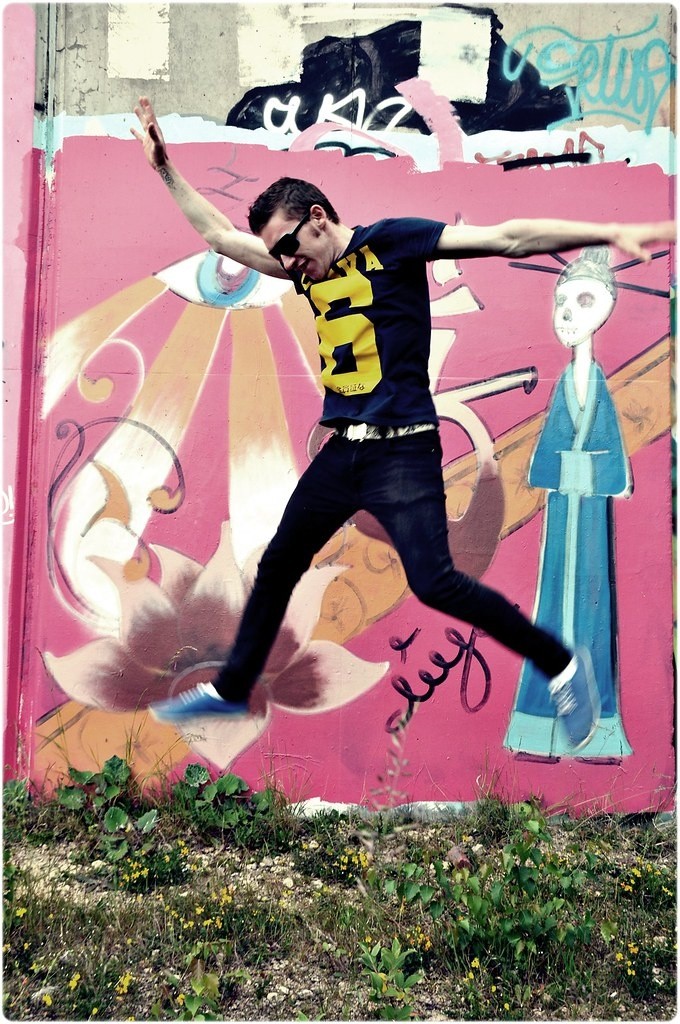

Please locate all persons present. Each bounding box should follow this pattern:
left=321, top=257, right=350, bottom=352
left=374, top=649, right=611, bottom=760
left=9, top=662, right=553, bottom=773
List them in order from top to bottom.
left=127, top=92, right=678, bottom=758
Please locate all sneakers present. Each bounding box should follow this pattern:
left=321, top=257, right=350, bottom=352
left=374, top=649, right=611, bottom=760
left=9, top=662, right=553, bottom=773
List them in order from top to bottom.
left=551, top=648, right=600, bottom=751
left=146, top=683, right=249, bottom=726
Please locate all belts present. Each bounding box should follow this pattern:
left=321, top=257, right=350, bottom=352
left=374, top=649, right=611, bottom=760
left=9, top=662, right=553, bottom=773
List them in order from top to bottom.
left=334, top=422, right=435, bottom=441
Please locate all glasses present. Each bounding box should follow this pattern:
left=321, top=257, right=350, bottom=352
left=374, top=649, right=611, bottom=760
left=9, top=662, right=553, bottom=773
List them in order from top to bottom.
left=268, top=211, right=310, bottom=260
